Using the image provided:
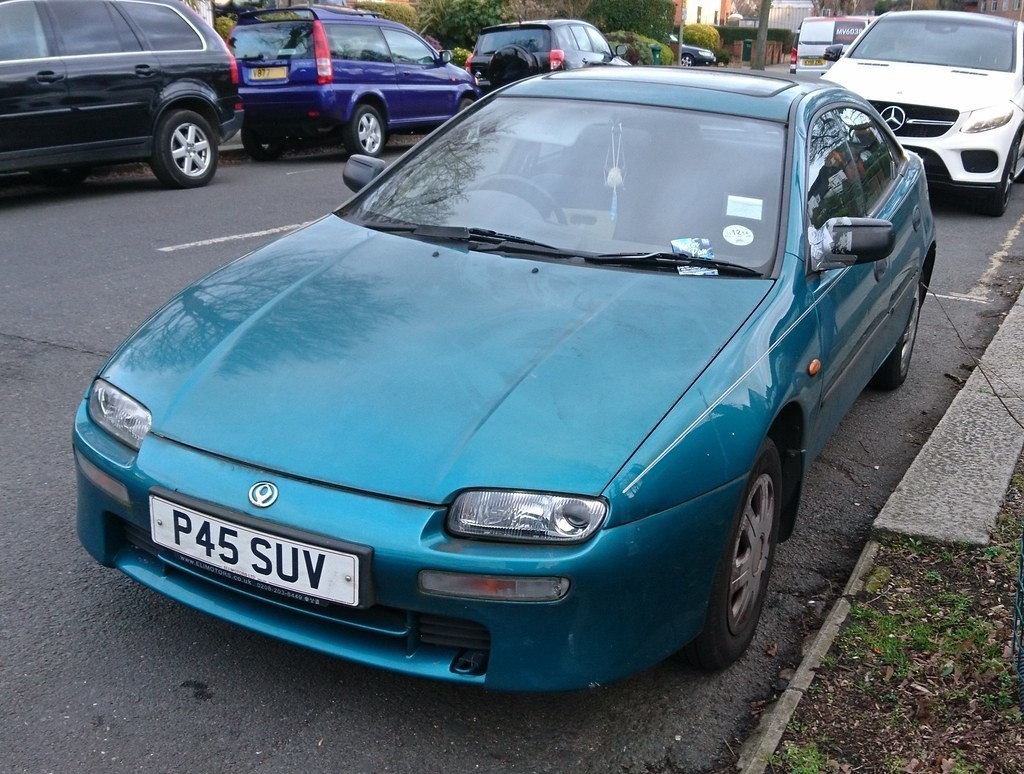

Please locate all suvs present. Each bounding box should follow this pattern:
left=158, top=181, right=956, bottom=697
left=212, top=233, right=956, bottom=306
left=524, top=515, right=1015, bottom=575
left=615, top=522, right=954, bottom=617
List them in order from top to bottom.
left=0, top=0, right=245, bottom=189
left=226, top=5, right=486, bottom=161
left=465, top=18, right=633, bottom=95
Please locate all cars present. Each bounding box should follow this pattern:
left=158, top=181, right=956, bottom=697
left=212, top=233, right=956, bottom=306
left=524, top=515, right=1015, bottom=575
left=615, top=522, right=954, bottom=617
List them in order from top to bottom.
left=790, top=11, right=1024, bottom=217
left=667, top=35, right=716, bottom=67
left=73, top=67, right=937, bottom=694
